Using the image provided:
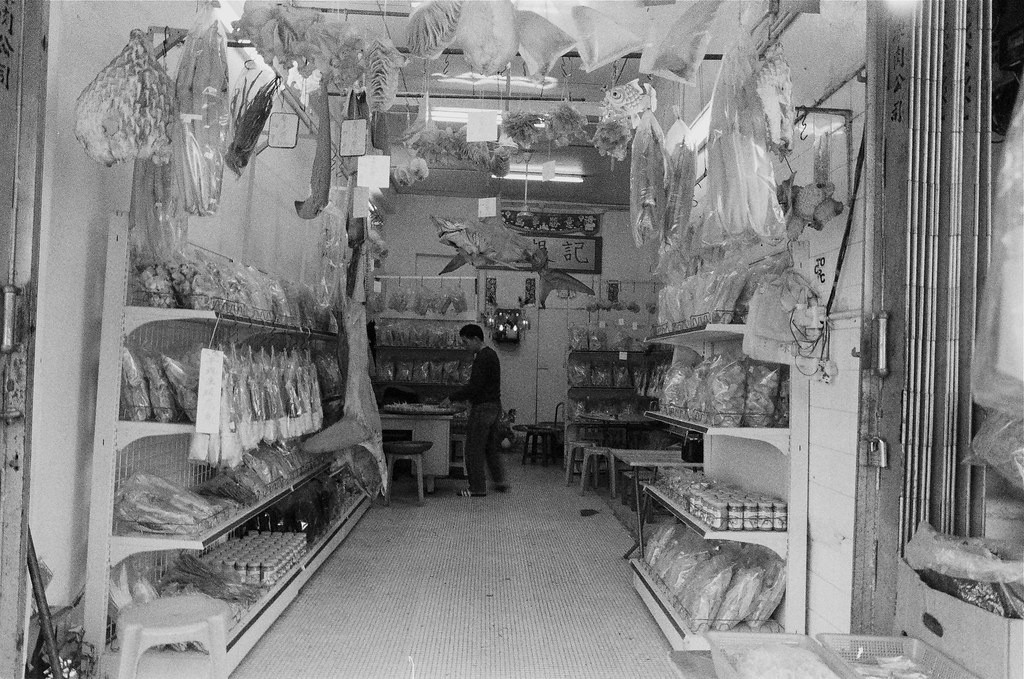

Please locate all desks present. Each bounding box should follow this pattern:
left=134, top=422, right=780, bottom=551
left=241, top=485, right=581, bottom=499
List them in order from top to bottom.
left=378, top=413, right=453, bottom=495
left=609, top=449, right=705, bottom=563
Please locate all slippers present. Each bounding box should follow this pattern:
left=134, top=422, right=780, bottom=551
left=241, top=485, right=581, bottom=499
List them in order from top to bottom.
left=457, top=489, right=488, bottom=497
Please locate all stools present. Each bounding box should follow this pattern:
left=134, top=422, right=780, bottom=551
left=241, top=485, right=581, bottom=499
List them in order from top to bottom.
left=522, top=431, right=555, bottom=468
left=451, top=433, right=468, bottom=477
left=564, top=440, right=599, bottom=489
left=578, top=446, right=618, bottom=498
left=384, top=453, right=425, bottom=506
left=622, top=471, right=654, bottom=511
left=114, top=594, right=229, bottom=679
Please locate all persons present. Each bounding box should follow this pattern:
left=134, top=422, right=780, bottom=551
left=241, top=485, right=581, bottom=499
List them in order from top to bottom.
left=438, top=324, right=509, bottom=497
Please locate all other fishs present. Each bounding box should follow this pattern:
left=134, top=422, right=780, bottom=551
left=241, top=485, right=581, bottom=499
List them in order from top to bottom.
left=606, top=77, right=658, bottom=129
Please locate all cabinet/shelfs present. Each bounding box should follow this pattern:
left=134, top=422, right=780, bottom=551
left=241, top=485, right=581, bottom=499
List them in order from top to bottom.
left=82, top=208, right=373, bottom=678
left=625, top=236, right=811, bottom=655
left=375, top=344, right=473, bottom=430
left=565, top=350, right=671, bottom=475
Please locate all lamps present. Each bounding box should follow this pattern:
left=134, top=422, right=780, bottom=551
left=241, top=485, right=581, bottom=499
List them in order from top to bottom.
left=492, top=172, right=586, bottom=182
